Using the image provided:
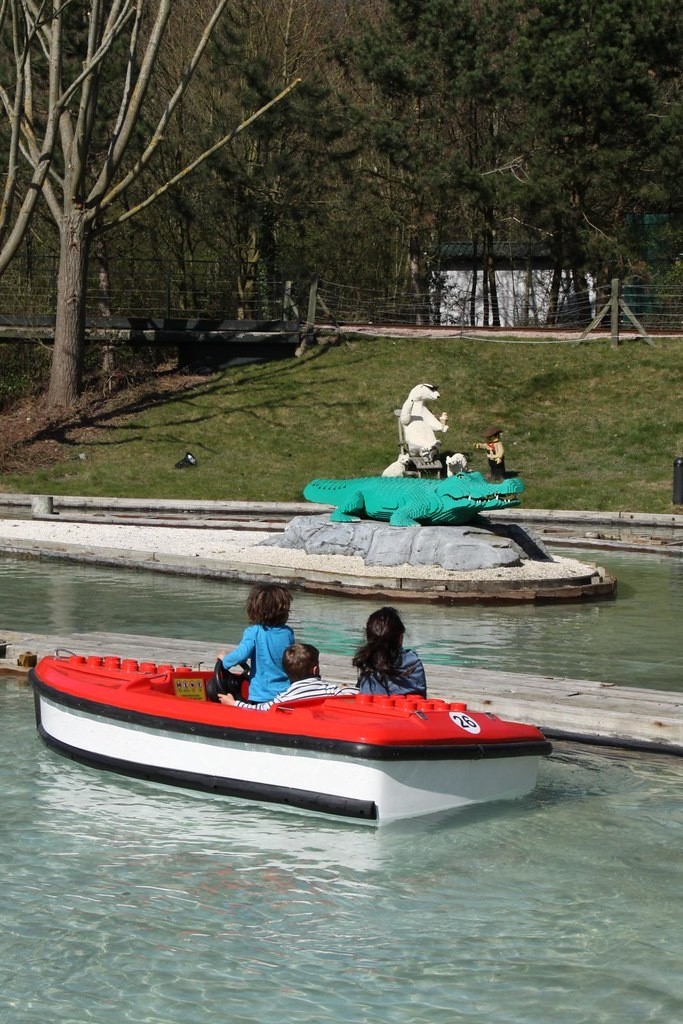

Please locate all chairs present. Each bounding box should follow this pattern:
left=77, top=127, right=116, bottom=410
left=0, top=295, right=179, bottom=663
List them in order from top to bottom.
left=394, top=410, right=442, bottom=479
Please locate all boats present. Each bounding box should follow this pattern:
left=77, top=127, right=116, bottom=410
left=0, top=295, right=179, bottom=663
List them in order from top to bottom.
left=28, top=647, right=558, bottom=831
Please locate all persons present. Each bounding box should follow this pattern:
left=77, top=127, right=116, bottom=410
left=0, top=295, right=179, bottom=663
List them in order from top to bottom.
left=472, top=427, right=506, bottom=483
left=216, top=583, right=295, bottom=705
left=350, top=607, right=427, bottom=699
left=217, top=643, right=359, bottom=710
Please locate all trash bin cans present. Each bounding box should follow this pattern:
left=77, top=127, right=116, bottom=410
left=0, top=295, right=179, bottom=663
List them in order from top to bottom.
left=673, top=457, right=683, bottom=504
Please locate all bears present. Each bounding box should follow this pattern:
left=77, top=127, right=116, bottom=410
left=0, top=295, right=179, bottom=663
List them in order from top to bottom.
left=382, top=453, right=410, bottom=477
